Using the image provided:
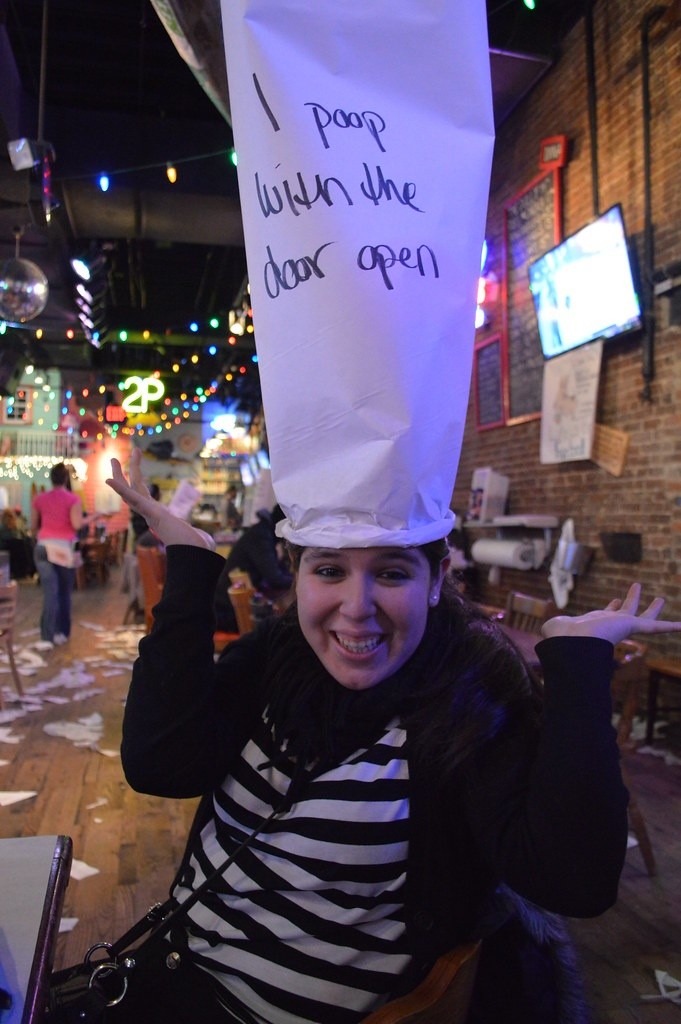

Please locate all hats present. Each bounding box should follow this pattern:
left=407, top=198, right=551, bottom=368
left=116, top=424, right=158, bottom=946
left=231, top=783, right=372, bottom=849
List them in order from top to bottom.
left=216, top=0, right=496, bottom=550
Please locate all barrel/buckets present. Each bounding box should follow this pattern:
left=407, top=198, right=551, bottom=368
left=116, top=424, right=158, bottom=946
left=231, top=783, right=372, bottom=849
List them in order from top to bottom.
left=557, top=539, right=598, bottom=576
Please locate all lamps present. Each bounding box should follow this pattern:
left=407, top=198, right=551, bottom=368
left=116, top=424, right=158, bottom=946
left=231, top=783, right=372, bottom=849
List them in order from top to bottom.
left=7, top=139, right=45, bottom=171
left=0, top=226, right=49, bottom=323
left=69, top=248, right=105, bottom=282
left=75, top=277, right=105, bottom=304
left=75, top=297, right=105, bottom=316
left=78, top=313, right=106, bottom=329
left=85, top=326, right=110, bottom=351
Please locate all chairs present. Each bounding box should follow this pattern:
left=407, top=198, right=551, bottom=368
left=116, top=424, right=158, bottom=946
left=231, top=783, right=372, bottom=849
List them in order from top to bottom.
left=136, top=544, right=166, bottom=638
left=226, top=580, right=257, bottom=636
left=0, top=581, right=24, bottom=713
left=471, top=591, right=658, bottom=877
left=645, top=657, right=681, bottom=745
left=359, top=907, right=514, bottom=1024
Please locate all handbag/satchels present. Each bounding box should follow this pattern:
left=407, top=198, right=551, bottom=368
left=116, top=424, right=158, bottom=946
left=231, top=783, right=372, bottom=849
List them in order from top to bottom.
left=32, top=940, right=172, bottom=1024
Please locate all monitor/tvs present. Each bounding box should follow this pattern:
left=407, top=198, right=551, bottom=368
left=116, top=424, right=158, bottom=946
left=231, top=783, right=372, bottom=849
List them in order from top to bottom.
left=527, top=201, right=644, bottom=361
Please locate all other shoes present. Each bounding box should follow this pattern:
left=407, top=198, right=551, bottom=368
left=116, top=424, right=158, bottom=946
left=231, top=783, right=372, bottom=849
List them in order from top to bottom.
left=134, top=614, right=145, bottom=624
left=54, top=633, right=67, bottom=645
left=36, top=640, right=53, bottom=652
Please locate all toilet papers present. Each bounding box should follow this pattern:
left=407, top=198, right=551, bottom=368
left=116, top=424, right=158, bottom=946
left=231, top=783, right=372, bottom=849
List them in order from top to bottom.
left=472, top=537, right=533, bottom=571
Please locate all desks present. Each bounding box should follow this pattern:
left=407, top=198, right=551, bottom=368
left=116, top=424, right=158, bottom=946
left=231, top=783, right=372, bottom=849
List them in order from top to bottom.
left=502, top=623, right=546, bottom=680
left=0, top=835, right=73, bottom=1024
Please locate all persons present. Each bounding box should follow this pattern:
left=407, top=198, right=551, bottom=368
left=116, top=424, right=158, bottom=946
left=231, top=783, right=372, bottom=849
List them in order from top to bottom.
left=105, top=449, right=681, bottom=1024
left=31, top=464, right=104, bottom=652
left=130, top=483, right=164, bottom=625
left=220, top=485, right=241, bottom=532
left=214, top=503, right=295, bottom=634
left=0, top=508, right=40, bottom=587
